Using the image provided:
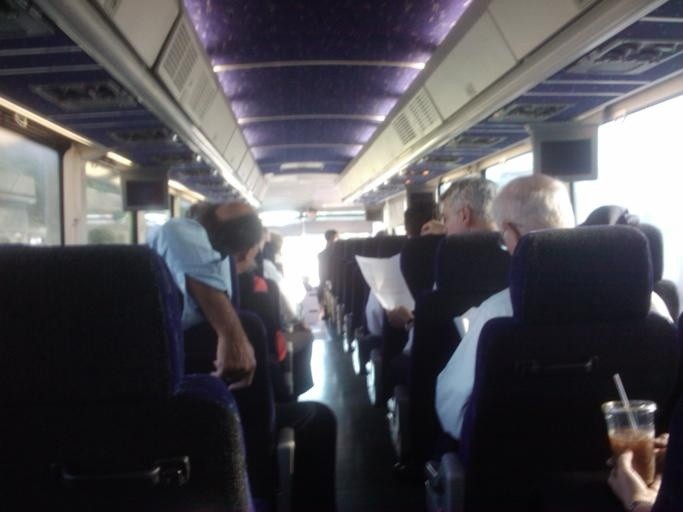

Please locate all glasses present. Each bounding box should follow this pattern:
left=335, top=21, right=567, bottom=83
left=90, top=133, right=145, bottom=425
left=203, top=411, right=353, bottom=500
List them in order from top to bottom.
left=496, top=226, right=508, bottom=251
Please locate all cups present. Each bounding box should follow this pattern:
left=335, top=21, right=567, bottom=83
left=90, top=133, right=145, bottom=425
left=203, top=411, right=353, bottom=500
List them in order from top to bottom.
left=604, top=400, right=657, bottom=487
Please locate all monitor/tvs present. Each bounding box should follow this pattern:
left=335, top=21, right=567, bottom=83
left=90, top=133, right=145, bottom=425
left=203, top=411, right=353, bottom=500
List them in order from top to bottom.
left=530, top=122, right=599, bottom=182
left=120, top=170, right=170, bottom=211
left=406, top=187, right=436, bottom=209
left=366, top=204, right=385, bottom=222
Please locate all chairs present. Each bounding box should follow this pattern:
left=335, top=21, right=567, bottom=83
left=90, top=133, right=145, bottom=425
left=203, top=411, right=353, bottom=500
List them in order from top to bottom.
left=324, top=237, right=683, bottom=512
left=2, top=244, right=295, bottom=511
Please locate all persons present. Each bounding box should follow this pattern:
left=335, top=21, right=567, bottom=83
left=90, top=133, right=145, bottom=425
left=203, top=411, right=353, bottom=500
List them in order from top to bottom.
left=436, top=175, right=683, bottom=512
left=366, top=199, right=441, bottom=352
left=150, top=201, right=338, bottom=512
left=317, top=230, right=339, bottom=320
